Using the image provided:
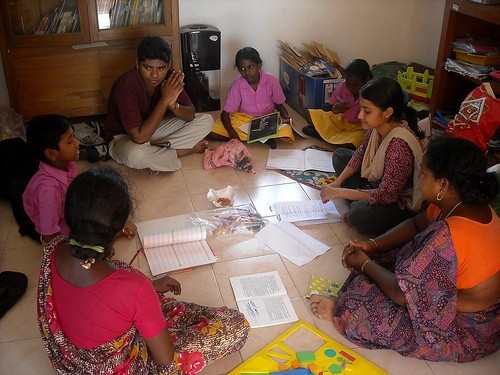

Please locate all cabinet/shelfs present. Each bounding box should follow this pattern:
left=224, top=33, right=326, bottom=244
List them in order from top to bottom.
left=0, top=0, right=184, bottom=124
left=429, top=0, right=500, bottom=178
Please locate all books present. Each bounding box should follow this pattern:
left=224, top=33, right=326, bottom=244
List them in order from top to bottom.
left=35, top=0, right=162, bottom=35
left=445, top=57, right=489, bottom=80
left=239, top=111, right=287, bottom=144
left=266, top=149, right=335, bottom=172
left=273, top=199, right=343, bottom=226
left=134, top=214, right=218, bottom=277
left=229, top=271, right=299, bottom=329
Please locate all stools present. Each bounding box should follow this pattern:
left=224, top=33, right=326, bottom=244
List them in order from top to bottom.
left=398, top=67, right=435, bottom=103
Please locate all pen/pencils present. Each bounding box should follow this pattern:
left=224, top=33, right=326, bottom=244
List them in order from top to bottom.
left=130, top=250, right=140, bottom=265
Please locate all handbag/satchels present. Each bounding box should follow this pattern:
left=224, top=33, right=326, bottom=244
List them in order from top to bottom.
left=444, top=82, right=500, bottom=155
left=71, top=119, right=114, bottom=161
left=204, top=139, right=256, bottom=174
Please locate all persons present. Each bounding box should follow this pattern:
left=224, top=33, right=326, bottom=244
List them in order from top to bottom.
left=106, top=36, right=214, bottom=176
left=207, top=47, right=293, bottom=149
left=301, top=58, right=374, bottom=152
left=320, top=76, right=428, bottom=235
left=21, top=115, right=138, bottom=245
left=310, top=136, right=500, bottom=363
left=37, top=166, right=252, bottom=375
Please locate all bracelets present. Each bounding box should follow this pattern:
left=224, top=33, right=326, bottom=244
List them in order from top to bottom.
left=332, top=103, right=339, bottom=113
left=369, top=238, right=378, bottom=252
left=361, top=260, right=374, bottom=276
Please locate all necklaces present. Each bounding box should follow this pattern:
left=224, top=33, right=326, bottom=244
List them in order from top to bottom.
left=438, top=201, right=462, bottom=219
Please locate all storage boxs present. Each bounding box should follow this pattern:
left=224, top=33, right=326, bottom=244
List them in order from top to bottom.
left=453, top=49, right=500, bottom=66
left=279, top=56, right=346, bottom=125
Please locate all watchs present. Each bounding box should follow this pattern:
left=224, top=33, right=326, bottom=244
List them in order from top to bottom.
left=169, top=102, right=179, bottom=111
left=284, top=116, right=292, bottom=125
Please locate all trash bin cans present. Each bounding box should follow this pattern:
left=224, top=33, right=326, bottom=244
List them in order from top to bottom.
left=180, top=24, right=223, bottom=112
